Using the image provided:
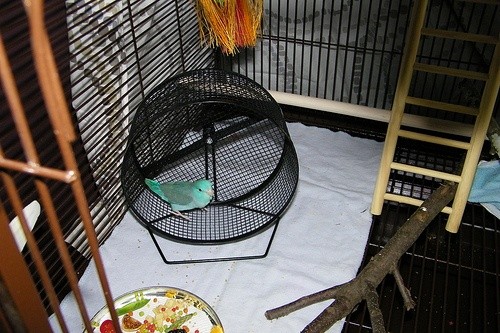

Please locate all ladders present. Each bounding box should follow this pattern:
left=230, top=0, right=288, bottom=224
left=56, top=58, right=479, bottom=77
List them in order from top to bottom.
left=371, top=0, right=500, bottom=233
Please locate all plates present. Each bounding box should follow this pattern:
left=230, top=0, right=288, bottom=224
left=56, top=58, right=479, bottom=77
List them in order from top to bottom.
left=83, top=286, right=224, bottom=333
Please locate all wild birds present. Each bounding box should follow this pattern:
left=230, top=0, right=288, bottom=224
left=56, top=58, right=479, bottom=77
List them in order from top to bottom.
left=144, top=177, right=214, bottom=222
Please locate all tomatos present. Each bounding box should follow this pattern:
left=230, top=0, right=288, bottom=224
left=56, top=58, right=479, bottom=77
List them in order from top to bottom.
left=100, top=319, right=115, bottom=332
